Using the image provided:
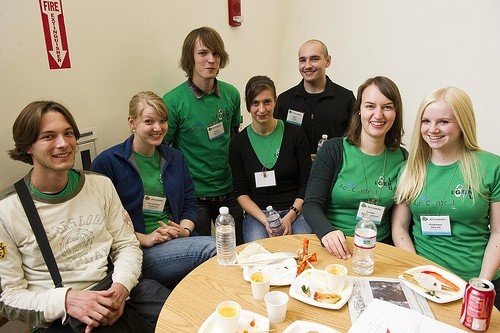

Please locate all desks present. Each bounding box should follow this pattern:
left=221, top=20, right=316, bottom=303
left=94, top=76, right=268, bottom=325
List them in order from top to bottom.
left=155, top=234, right=500, bottom=333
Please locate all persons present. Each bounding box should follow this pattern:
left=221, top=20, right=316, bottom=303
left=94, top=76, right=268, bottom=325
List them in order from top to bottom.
left=161, top=27, right=243, bottom=246
left=272, top=39, right=358, bottom=162
left=229, top=76, right=314, bottom=244
left=301, top=76, right=409, bottom=260
left=391, top=87, right=500, bottom=312
left=90, top=91, right=217, bottom=288
left=0, top=101, right=172, bottom=333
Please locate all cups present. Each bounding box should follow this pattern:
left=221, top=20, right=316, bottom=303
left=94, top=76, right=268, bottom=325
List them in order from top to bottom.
left=325, top=264, right=348, bottom=292
left=250, top=271, right=270, bottom=300
left=264, top=291, right=289, bottom=323
left=216, top=301, right=242, bottom=333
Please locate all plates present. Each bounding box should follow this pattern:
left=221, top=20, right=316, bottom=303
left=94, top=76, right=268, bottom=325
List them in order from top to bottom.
left=243, top=253, right=297, bottom=286
left=398, top=265, right=466, bottom=303
left=289, top=269, right=353, bottom=309
left=198, top=309, right=270, bottom=333
left=283, top=320, right=340, bottom=333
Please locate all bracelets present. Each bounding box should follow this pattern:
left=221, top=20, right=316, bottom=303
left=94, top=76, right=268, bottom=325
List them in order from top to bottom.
left=184, top=228, right=192, bottom=235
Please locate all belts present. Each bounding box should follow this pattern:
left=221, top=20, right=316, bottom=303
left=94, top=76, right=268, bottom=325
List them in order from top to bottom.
left=198, top=192, right=234, bottom=202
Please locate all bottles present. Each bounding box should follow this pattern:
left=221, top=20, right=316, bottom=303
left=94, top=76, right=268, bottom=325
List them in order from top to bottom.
left=317, top=134, right=328, bottom=151
left=266, top=206, right=285, bottom=237
left=215, top=207, right=237, bottom=265
left=353, top=212, right=377, bottom=275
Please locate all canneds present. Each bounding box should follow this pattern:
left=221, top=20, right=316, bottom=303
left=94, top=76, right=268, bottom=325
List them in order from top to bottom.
left=459, top=278, right=496, bottom=332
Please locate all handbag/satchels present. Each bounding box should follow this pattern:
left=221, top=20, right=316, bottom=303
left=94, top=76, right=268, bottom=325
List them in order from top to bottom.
left=66, top=271, right=139, bottom=333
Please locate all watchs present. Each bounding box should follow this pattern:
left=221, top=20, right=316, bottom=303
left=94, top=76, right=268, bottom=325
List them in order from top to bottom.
left=289, top=206, right=301, bottom=217
